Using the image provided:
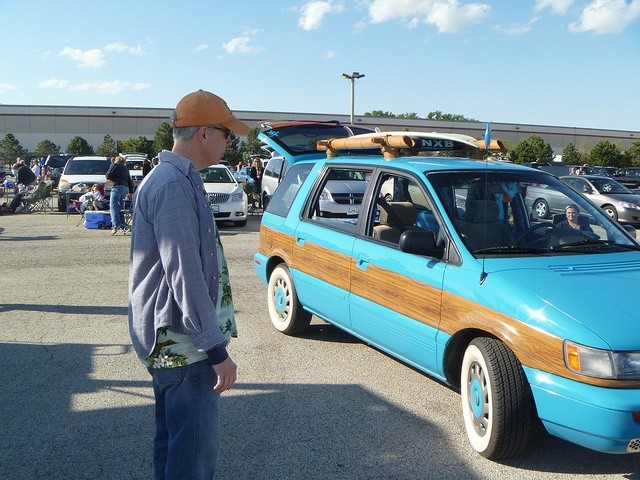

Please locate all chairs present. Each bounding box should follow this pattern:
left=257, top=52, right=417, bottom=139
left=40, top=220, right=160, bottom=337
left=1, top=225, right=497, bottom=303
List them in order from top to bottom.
left=39, top=180, right=53, bottom=211
left=21, top=182, right=45, bottom=214
left=458, top=198, right=519, bottom=253
left=70, top=199, right=86, bottom=227
left=372, top=202, right=424, bottom=245
left=120, top=207, right=134, bottom=235
left=552, top=212, right=590, bottom=227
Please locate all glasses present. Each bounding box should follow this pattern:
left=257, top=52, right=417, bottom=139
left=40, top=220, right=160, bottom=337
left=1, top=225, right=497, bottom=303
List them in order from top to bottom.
left=208, top=126, right=231, bottom=140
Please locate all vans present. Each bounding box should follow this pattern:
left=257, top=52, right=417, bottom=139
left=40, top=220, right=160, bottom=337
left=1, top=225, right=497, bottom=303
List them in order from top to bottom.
left=261, top=156, right=385, bottom=226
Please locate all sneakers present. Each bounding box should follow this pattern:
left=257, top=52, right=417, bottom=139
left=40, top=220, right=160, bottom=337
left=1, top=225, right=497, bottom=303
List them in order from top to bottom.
left=110, top=225, right=121, bottom=236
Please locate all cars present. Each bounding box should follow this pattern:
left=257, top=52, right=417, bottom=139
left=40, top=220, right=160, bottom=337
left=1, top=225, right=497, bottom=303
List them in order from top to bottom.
left=253, top=120, right=640, bottom=461
left=119, top=152, right=148, bottom=188
left=45, top=154, right=74, bottom=183
left=57, top=156, right=112, bottom=214
left=197, top=164, right=248, bottom=226
left=538, top=164, right=640, bottom=180
left=525, top=174, right=640, bottom=227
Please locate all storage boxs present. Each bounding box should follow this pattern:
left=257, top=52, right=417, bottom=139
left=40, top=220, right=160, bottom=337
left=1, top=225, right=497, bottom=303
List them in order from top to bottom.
left=4, top=180, right=15, bottom=188
left=84, top=211, right=111, bottom=229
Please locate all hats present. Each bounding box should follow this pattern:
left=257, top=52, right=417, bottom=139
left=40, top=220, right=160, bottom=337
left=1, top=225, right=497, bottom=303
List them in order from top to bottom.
left=171, top=89, right=253, bottom=137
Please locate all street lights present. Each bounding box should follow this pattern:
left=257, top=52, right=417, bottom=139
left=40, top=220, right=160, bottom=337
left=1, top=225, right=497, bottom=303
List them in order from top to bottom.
left=342, top=71, right=365, bottom=124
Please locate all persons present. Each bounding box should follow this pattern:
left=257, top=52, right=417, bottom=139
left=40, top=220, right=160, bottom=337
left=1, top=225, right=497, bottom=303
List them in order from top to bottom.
left=128, top=90, right=253, bottom=479
left=106, top=156, right=134, bottom=236
left=13, top=158, right=20, bottom=167
left=151, top=158, right=159, bottom=168
left=250, top=158, right=264, bottom=209
left=143, top=159, right=152, bottom=177
left=20, top=160, right=25, bottom=165
left=31, top=160, right=40, bottom=177
left=244, top=160, right=252, bottom=181
left=236, top=161, right=246, bottom=175
left=12, top=162, right=37, bottom=187
left=576, top=166, right=586, bottom=175
left=569, top=167, right=576, bottom=176
left=79, top=182, right=105, bottom=213
left=556, top=204, right=594, bottom=232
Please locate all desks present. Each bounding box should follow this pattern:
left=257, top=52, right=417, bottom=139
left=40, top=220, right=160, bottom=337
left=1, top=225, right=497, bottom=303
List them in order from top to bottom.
left=5, top=187, right=17, bottom=203
left=66, top=190, right=87, bottom=219
